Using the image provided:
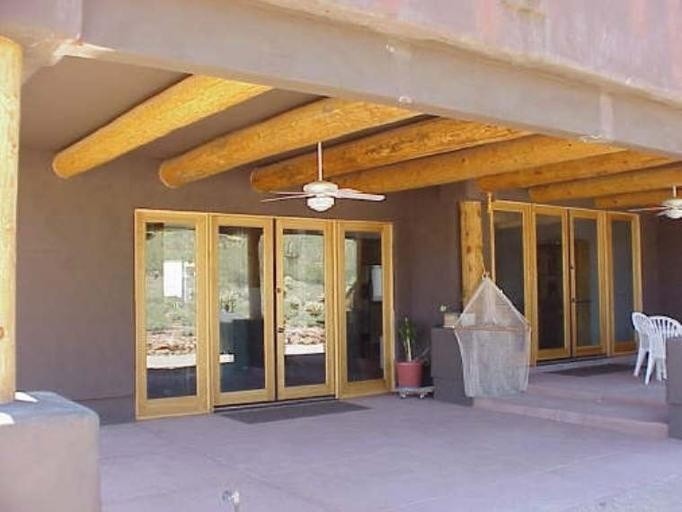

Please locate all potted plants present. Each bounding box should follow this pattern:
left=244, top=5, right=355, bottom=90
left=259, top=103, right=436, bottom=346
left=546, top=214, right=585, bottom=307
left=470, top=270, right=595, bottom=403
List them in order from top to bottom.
left=394, top=312, right=430, bottom=388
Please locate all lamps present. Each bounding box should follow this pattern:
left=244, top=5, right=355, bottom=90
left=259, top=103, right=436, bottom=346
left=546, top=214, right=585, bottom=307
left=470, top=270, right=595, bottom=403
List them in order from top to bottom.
left=304, top=198, right=334, bottom=212
left=664, top=210, right=682, bottom=219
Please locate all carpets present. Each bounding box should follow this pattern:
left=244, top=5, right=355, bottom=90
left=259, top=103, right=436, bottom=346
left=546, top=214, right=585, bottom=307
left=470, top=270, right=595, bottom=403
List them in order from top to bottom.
left=544, top=362, right=648, bottom=377
left=221, top=401, right=371, bottom=424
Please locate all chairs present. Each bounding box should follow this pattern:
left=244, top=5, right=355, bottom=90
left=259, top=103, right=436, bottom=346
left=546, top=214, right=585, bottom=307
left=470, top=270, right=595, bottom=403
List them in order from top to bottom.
left=632, top=312, right=682, bottom=385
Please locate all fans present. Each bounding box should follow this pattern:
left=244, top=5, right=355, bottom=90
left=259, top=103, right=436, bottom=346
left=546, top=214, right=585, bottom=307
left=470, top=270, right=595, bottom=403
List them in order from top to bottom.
left=260, top=141, right=387, bottom=203
left=627, top=187, right=682, bottom=216
